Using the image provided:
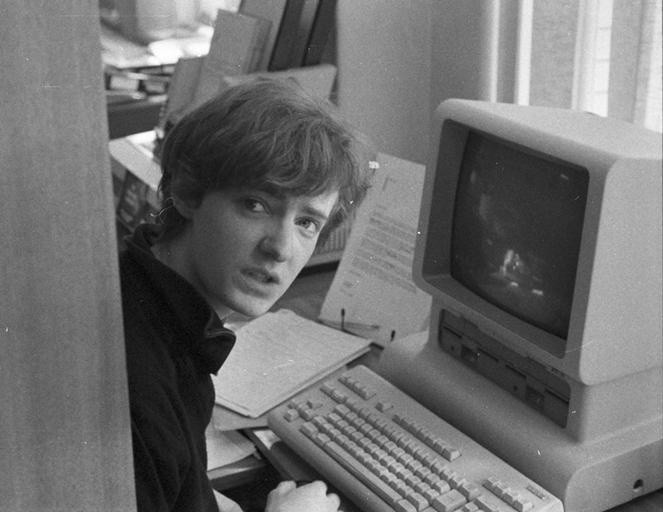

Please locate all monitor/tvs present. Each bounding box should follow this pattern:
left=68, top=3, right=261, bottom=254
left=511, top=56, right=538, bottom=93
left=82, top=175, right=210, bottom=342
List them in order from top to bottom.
left=374, top=97, right=663, bottom=511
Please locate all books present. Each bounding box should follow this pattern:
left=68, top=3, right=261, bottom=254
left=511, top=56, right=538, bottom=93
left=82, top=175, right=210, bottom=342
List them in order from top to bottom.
left=156, top=1, right=336, bottom=130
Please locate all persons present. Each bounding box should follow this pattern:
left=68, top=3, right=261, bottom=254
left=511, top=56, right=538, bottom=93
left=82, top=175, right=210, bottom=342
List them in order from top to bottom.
left=117, top=77, right=361, bottom=512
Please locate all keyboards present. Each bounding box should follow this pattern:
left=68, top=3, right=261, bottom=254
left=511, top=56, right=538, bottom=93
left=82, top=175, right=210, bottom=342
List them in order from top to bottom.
left=268, top=364, right=565, bottom=512
